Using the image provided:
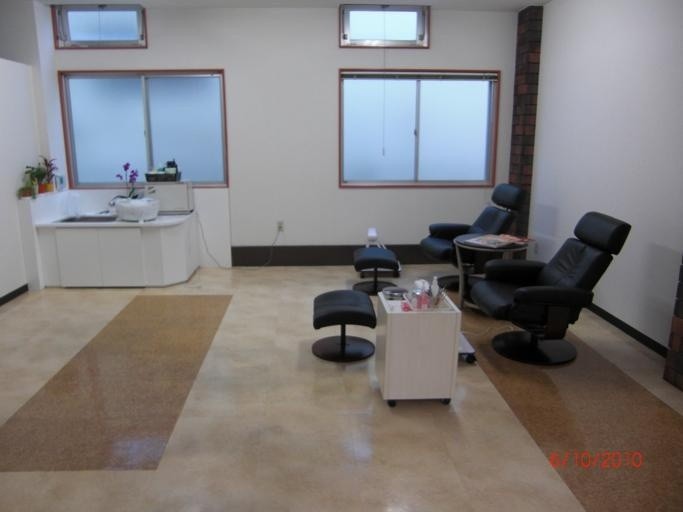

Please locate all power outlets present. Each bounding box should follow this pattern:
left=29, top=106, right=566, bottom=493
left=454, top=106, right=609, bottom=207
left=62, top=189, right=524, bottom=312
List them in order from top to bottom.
left=276, top=221, right=284, bottom=233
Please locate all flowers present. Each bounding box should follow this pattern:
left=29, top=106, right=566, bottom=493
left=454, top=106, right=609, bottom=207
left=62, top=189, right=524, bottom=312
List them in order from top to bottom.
left=109, top=161, right=140, bottom=202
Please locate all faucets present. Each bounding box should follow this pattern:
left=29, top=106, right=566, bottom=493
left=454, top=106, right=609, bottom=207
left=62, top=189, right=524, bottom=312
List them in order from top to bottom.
left=108, top=194, right=128, bottom=207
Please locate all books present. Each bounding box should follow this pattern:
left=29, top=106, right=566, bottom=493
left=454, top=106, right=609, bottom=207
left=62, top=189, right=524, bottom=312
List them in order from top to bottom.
left=498, top=232, right=535, bottom=245
left=464, top=234, right=513, bottom=249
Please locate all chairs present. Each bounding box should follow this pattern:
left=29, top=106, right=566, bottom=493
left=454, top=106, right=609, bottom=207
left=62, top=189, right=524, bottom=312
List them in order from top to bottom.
left=418, top=182, right=525, bottom=293
left=469, top=210, right=631, bottom=367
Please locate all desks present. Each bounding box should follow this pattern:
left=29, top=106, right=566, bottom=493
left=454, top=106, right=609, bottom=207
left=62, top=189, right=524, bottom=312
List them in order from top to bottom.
left=452, top=231, right=538, bottom=312
left=374, top=286, right=463, bottom=408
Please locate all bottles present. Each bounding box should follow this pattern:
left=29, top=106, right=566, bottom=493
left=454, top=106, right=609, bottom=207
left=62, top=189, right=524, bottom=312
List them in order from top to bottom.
left=148, top=161, right=176, bottom=174
left=31, top=181, right=38, bottom=200
left=414, top=275, right=440, bottom=309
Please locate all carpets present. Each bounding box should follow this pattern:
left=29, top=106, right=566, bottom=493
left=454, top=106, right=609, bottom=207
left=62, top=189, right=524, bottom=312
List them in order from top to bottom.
left=0, top=294, right=235, bottom=474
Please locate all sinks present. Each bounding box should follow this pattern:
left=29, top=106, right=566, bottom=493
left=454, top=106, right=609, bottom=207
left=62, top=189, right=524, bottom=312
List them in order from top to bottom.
left=53, top=215, right=120, bottom=222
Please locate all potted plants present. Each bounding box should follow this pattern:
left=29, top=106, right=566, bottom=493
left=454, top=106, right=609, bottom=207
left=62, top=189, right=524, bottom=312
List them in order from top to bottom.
left=13, top=153, right=59, bottom=202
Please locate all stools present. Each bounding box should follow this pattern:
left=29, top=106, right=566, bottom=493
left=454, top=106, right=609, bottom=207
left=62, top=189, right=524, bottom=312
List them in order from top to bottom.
left=350, top=244, right=401, bottom=296
left=309, top=286, right=378, bottom=366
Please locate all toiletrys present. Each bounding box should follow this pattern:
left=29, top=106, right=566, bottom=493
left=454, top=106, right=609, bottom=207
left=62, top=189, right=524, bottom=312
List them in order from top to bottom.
left=430, top=276, right=439, bottom=298
left=417, top=281, right=429, bottom=310
